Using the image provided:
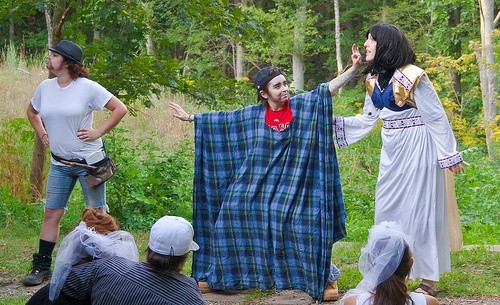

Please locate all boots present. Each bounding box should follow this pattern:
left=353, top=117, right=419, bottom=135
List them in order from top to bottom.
left=23, top=254, right=51, bottom=284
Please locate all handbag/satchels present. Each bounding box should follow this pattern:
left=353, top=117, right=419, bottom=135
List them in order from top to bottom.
left=85, top=158, right=116, bottom=188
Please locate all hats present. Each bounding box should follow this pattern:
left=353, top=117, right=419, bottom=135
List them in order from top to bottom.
left=48, top=40, right=83, bottom=66
left=253, top=67, right=283, bottom=103
left=148, top=215, right=199, bottom=255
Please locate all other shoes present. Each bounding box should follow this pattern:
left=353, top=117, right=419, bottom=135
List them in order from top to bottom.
left=322, top=278, right=338, bottom=300
left=197, top=280, right=212, bottom=293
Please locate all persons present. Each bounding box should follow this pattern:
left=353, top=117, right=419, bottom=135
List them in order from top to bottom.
left=327, top=24, right=466, bottom=298
left=25, top=40, right=128, bottom=286
left=168, top=43, right=362, bottom=301
left=24, top=207, right=119, bottom=305
left=59, top=215, right=206, bottom=304
left=335, top=221, right=440, bottom=305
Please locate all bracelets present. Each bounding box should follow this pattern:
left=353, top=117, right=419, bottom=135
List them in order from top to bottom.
left=188, top=115, right=192, bottom=124
left=40, top=133, right=48, bottom=142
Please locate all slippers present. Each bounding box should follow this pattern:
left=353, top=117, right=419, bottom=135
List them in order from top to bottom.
left=412, top=283, right=438, bottom=296
